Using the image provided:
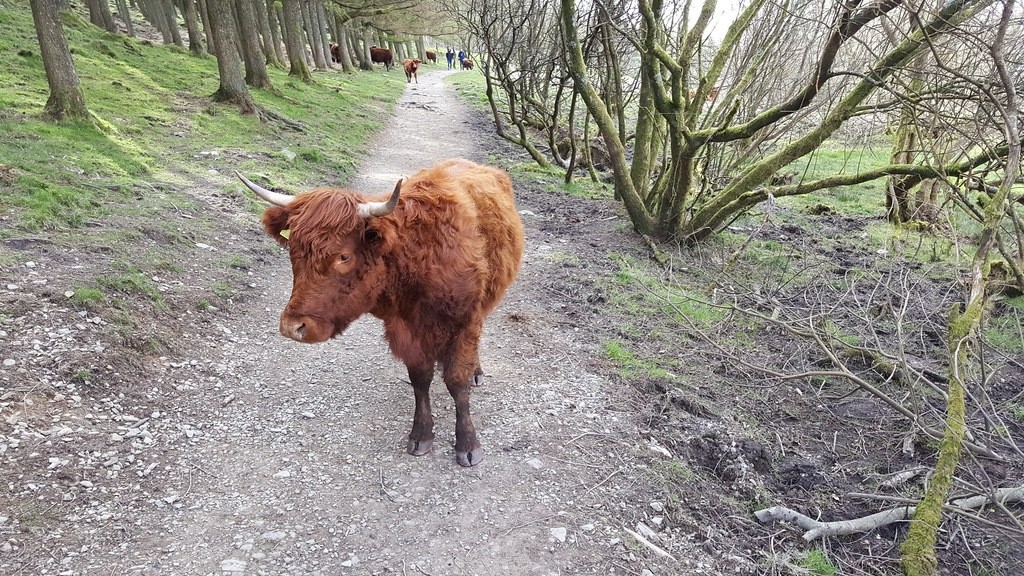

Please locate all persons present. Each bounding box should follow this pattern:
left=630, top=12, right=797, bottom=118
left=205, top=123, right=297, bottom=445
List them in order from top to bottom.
left=446, top=48, right=454, bottom=70
left=458, top=50, right=465, bottom=70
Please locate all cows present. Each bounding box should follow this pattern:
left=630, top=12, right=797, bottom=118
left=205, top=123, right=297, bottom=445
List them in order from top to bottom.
left=329, top=42, right=341, bottom=64
left=370, top=45, right=393, bottom=72
left=426, top=50, right=436, bottom=64
left=403, top=59, right=422, bottom=83
left=458, top=59, right=474, bottom=70
left=234, top=158, right=524, bottom=467
